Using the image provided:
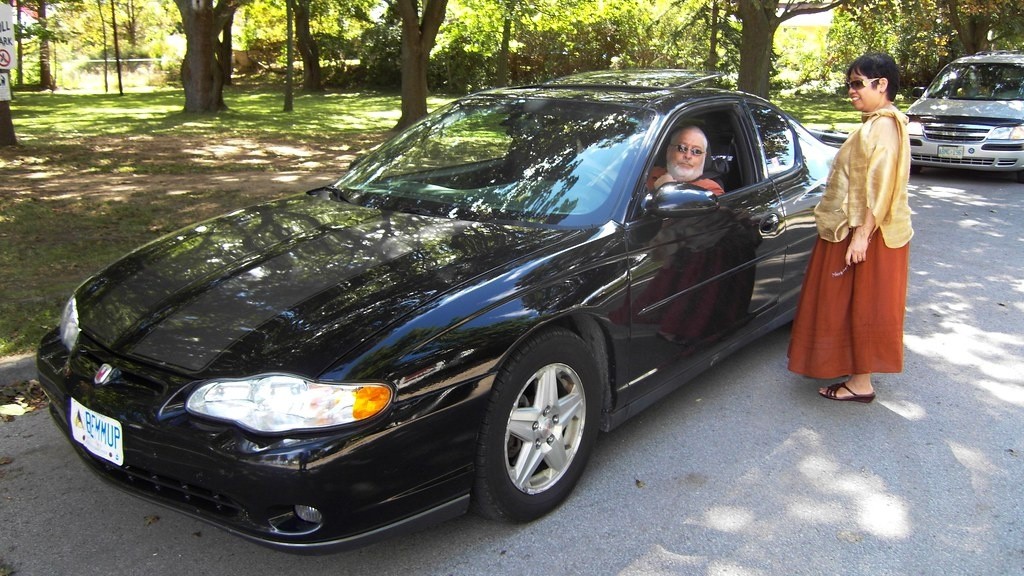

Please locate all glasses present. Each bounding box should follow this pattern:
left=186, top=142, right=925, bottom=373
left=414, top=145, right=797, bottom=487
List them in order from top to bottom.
left=846, top=78, right=879, bottom=91
left=672, top=145, right=706, bottom=156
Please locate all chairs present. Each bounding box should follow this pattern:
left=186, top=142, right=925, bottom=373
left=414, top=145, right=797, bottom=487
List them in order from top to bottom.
left=656, top=125, right=724, bottom=191
left=512, top=142, right=577, bottom=184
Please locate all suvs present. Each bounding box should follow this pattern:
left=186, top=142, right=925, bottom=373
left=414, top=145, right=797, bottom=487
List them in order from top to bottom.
left=904, top=52, right=1024, bottom=182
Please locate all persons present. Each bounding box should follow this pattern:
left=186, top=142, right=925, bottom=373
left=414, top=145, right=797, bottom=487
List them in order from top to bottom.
left=788, top=52, right=914, bottom=404
left=576, top=126, right=725, bottom=197
left=607, top=192, right=763, bottom=346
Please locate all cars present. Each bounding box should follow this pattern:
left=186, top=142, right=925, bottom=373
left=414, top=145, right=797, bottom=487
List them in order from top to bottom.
left=36, top=70, right=841, bottom=556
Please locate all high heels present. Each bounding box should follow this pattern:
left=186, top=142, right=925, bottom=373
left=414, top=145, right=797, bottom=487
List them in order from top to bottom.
left=819, top=382, right=876, bottom=403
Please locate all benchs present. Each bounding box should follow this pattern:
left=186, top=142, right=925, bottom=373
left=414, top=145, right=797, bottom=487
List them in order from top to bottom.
left=710, top=134, right=743, bottom=190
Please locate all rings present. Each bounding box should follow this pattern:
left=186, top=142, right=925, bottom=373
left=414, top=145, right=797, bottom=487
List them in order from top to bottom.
left=858, top=257, right=862, bottom=258
